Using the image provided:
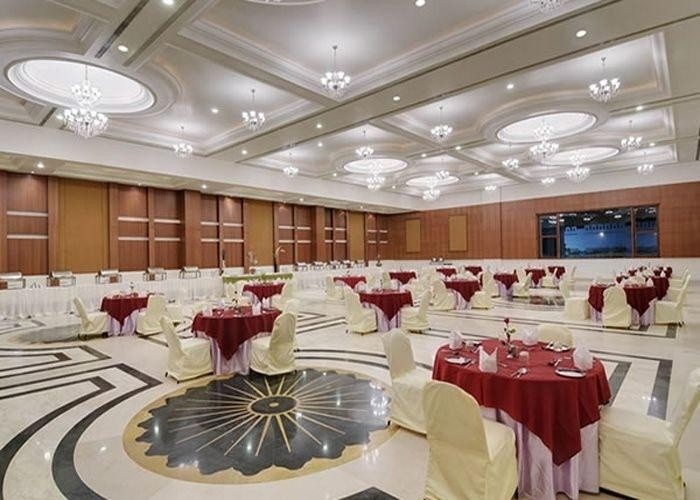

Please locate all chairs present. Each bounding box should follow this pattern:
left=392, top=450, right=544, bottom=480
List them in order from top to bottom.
left=0, top=258, right=694, bottom=384
left=381, top=327, right=433, bottom=435
left=599, top=367, right=700, bottom=500
left=422, top=378, right=519, bottom=500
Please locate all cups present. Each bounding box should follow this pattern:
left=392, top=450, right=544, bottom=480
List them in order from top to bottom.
left=553, top=341, right=562, bottom=353
left=465, top=342, right=474, bottom=354
left=520, top=351, right=529, bottom=370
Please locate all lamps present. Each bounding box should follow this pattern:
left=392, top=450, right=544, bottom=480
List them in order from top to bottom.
left=320, top=43, right=353, bottom=97
left=587, top=55, right=624, bottom=104
left=60, top=65, right=111, bottom=141
left=240, top=87, right=266, bottom=132
left=430, top=105, right=454, bottom=142
left=481, top=115, right=592, bottom=193
left=620, top=118, right=643, bottom=152
left=172, top=124, right=194, bottom=159
left=356, top=129, right=374, bottom=159
left=365, top=141, right=522, bottom=204
left=636, top=151, right=655, bottom=177
left=283, top=152, right=299, bottom=179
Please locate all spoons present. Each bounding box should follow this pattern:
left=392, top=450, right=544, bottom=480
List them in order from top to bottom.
left=554, top=356, right=563, bottom=368
left=517, top=368, right=527, bottom=378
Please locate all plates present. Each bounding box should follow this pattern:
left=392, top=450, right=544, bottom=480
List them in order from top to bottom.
left=464, top=340, right=482, bottom=344
left=546, top=342, right=570, bottom=352
left=444, top=355, right=469, bottom=365
left=555, top=367, right=586, bottom=377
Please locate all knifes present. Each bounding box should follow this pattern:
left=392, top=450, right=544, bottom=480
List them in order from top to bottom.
left=559, top=368, right=584, bottom=373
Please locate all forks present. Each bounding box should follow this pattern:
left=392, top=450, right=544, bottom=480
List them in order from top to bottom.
left=463, top=358, right=476, bottom=369
